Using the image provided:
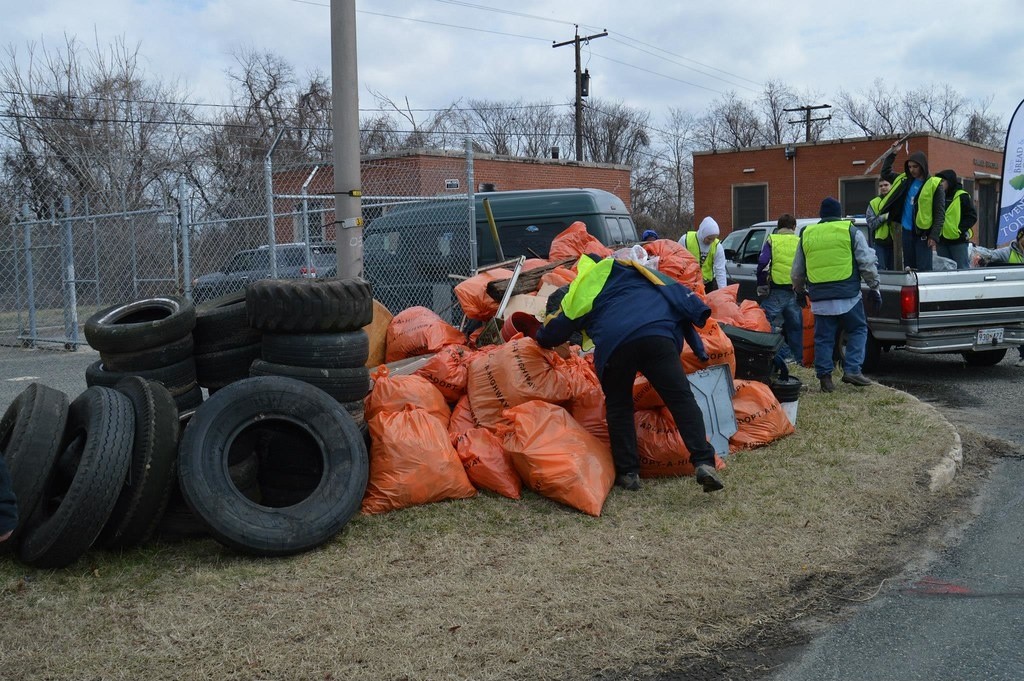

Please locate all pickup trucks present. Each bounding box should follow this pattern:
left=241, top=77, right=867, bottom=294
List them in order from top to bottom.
left=720, top=217, right=1024, bottom=374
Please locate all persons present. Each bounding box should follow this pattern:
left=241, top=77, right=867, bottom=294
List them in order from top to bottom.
left=878, top=140, right=945, bottom=271
left=935, top=169, right=977, bottom=269
left=867, top=179, right=893, bottom=270
left=791, top=197, right=882, bottom=392
left=757, top=213, right=804, bottom=364
left=679, top=217, right=727, bottom=294
left=981, top=228, right=1024, bottom=366
left=642, top=230, right=659, bottom=241
left=536, top=255, right=724, bottom=492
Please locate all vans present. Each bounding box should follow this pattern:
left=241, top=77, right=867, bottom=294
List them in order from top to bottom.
left=319, top=189, right=639, bottom=333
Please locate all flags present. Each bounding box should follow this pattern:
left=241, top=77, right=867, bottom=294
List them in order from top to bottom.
left=997, top=102, right=1024, bottom=245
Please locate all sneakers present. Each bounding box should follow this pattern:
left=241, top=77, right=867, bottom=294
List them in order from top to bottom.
left=1014, top=358, right=1024, bottom=367
left=842, top=372, right=870, bottom=387
left=820, top=375, right=834, bottom=393
left=695, top=465, right=724, bottom=493
left=619, top=473, right=640, bottom=491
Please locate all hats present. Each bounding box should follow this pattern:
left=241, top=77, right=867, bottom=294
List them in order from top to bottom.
left=1016, top=227, right=1024, bottom=242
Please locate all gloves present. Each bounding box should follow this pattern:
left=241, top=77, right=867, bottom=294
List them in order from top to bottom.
left=757, top=285, right=770, bottom=297
left=868, top=288, right=883, bottom=316
left=796, top=289, right=808, bottom=307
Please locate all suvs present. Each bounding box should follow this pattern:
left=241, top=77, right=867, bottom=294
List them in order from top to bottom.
left=192, top=242, right=338, bottom=307
